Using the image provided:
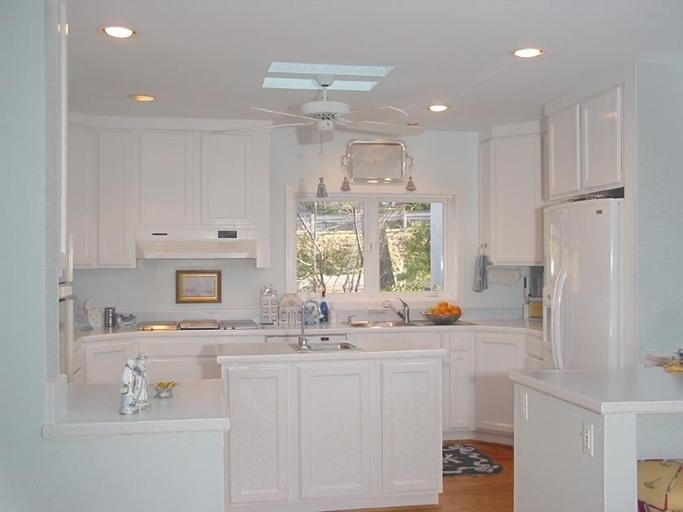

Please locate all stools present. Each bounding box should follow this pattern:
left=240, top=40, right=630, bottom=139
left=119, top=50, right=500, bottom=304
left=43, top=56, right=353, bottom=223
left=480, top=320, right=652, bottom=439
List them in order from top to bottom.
left=636, top=458, right=683, bottom=511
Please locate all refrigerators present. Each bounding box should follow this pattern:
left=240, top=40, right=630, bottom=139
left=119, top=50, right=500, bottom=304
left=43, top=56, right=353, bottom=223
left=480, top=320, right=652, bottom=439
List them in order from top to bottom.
left=537, top=196, right=627, bottom=375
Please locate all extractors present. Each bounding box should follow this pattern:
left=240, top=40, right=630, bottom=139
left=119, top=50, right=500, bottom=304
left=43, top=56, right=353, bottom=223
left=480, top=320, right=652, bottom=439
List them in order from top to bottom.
left=135, top=226, right=256, bottom=258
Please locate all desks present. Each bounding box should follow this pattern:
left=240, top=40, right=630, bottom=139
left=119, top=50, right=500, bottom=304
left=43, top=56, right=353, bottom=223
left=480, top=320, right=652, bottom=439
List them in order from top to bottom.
left=505, top=366, right=683, bottom=512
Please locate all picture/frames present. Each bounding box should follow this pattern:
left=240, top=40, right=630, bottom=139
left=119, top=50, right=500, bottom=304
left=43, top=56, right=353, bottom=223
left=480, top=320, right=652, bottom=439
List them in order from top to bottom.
left=174, top=269, right=222, bottom=303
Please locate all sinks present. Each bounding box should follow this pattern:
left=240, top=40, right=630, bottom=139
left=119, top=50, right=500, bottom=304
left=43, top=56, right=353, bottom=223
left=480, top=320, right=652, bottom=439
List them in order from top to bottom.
left=351, top=320, right=419, bottom=328
left=287, top=341, right=359, bottom=353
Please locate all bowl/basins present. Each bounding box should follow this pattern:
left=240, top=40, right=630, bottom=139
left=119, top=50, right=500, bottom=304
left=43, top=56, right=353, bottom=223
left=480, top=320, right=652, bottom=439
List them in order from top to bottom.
left=422, top=311, right=465, bottom=326
left=116, top=318, right=135, bottom=328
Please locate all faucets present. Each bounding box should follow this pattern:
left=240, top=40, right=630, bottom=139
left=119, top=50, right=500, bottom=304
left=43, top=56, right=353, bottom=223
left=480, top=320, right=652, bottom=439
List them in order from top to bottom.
left=297, top=298, right=321, bottom=350
left=383, top=299, right=406, bottom=322
left=347, top=314, right=355, bottom=324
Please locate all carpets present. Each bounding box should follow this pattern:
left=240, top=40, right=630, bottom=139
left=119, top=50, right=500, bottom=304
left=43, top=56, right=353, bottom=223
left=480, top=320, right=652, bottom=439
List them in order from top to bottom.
left=441, top=442, right=503, bottom=477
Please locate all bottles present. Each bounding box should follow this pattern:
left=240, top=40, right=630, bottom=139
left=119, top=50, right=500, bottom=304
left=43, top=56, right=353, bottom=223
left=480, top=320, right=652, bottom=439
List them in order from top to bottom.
left=319, top=290, right=328, bottom=323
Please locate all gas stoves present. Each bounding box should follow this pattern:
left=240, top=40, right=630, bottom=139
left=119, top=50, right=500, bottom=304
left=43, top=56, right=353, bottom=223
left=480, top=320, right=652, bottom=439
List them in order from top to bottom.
left=136, top=319, right=259, bottom=332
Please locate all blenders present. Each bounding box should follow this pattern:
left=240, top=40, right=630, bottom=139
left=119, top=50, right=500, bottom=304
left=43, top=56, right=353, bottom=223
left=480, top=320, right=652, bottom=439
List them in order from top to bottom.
left=529, top=266, right=544, bottom=322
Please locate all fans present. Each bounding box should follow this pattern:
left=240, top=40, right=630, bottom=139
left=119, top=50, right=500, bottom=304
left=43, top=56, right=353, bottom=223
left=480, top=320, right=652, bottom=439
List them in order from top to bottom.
left=233, top=74, right=424, bottom=137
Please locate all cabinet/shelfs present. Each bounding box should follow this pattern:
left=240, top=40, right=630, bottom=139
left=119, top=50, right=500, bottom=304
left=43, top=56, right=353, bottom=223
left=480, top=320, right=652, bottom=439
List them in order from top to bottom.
left=547, top=88, right=622, bottom=199
left=66, top=122, right=98, bottom=266
left=98, top=130, right=136, bottom=266
left=136, top=131, right=195, bottom=227
left=195, top=132, right=253, bottom=227
left=478, top=133, right=543, bottom=264
left=441, top=333, right=473, bottom=430
left=473, top=334, right=524, bottom=433
left=524, top=337, right=555, bottom=371
left=138, top=342, right=222, bottom=381
left=86, top=343, right=138, bottom=384
left=70, top=344, right=86, bottom=385
left=378, top=360, right=439, bottom=493
left=297, top=363, right=373, bottom=497
left=225, top=365, right=290, bottom=503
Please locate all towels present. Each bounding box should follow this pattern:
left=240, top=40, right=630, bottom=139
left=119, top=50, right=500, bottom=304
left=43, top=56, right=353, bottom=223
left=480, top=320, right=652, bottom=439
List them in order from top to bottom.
left=470, top=255, right=488, bottom=292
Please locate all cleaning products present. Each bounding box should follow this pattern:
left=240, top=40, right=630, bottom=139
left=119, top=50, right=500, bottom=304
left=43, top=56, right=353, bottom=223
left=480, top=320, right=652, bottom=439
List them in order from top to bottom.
left=258, top=281, right=279, bottom=326
left=319, top=289, right=330, bottom=323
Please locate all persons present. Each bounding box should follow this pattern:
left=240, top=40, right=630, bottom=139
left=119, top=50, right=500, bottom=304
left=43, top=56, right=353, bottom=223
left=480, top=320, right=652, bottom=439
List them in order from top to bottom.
left=118, top=353, right=149, bottom=415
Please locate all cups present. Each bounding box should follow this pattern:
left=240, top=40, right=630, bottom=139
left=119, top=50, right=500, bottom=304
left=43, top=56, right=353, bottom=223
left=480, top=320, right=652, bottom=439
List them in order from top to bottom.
left=104, top=307, right=115, bottom=327
left=153, top=385, right=175, bottom=400
